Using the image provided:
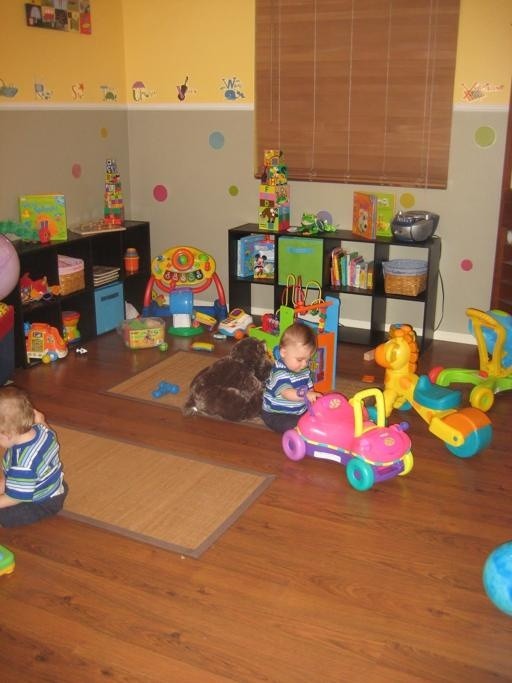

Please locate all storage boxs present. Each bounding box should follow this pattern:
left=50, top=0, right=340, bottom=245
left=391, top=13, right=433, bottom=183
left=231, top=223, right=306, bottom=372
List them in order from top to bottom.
left=93, top=281, right=126, bottom=336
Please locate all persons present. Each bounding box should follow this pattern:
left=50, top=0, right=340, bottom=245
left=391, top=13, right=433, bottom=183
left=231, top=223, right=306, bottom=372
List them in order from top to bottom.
left=262, top=320, right=325, bottom=433
left=0, top=381, right=70, bottom=529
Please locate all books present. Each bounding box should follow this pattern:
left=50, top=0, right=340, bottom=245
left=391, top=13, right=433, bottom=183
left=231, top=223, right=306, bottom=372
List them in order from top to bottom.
left=352, top=190, right=378, bottom=241
left=366, top=191, right=397, bottom=238
left=236, top=233, right=274, bottom=279
left=331, top=246, right=373, bottom=291
left=93, top=265, right=121, bottom=287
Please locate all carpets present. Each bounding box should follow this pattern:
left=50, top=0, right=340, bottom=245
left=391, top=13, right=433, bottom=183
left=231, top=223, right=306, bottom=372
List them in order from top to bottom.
left=92, top=346, right=278, bottom=432
left=0, top=419, right=278, bottom=562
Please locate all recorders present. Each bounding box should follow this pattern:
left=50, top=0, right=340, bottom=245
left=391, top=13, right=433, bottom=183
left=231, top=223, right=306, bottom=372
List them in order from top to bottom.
left=391, top=210, right=440, bottom=242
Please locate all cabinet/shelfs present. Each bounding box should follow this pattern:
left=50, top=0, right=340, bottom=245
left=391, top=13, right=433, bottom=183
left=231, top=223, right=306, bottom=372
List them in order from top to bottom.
left=0, top=218, right=153, bottom=368
left=228, top=222, right=442, bottom=356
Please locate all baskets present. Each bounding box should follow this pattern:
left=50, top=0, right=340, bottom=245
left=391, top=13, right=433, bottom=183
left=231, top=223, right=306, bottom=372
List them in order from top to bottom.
left=58, top=254, right=86, bottom=295
left=382, top=259, right=428, bottom=297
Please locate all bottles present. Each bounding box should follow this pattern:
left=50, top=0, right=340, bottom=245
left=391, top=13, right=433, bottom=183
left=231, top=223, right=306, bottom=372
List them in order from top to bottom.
left=124, top=246, right=139, bottom=273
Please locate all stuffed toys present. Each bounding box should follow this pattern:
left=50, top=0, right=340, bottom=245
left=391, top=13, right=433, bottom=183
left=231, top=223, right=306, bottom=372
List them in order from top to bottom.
left=179, top=336, right=272, bottom=424
